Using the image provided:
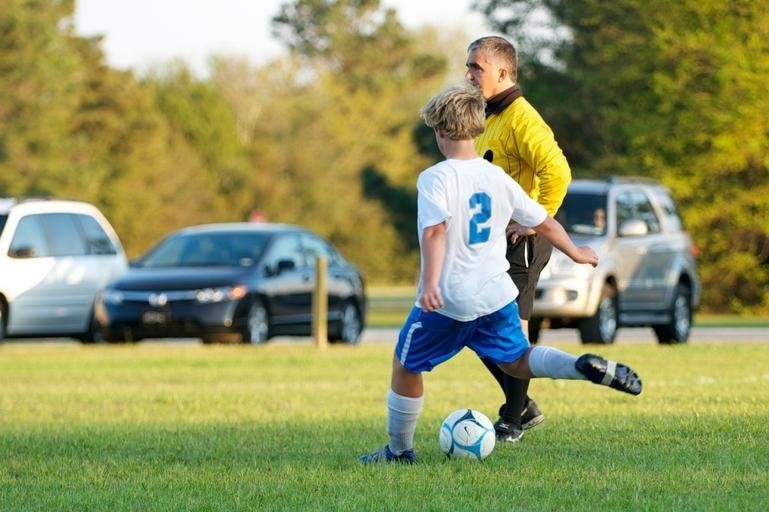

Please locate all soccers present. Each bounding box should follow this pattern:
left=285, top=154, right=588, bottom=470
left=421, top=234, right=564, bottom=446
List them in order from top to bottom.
left=439, top=408, right=496, bottom=459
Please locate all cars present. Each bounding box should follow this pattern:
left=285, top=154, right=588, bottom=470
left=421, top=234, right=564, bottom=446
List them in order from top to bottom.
left=90, top=221, right=366, bottom=346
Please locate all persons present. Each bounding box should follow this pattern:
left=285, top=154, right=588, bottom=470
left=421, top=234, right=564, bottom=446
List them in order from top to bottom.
left=461, top=36, right=571, bottom=445
left=357, top=84, right=641, bottom=465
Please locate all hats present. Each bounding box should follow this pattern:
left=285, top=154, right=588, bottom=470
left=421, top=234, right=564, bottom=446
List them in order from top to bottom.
left=575, top=355, right=644, bottom=397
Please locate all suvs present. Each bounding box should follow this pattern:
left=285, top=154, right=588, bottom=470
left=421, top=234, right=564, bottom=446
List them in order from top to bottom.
left=525, top=171, right=701, bottom=344
left=1, top=197, right=131, bottom=345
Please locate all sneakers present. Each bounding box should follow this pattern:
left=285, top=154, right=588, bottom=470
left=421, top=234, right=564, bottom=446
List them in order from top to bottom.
left=498, top=402, right=547, bottom=429
left=494, top=418, right=528, bottom=444
left=360, top=446, right=417, bottom=465
